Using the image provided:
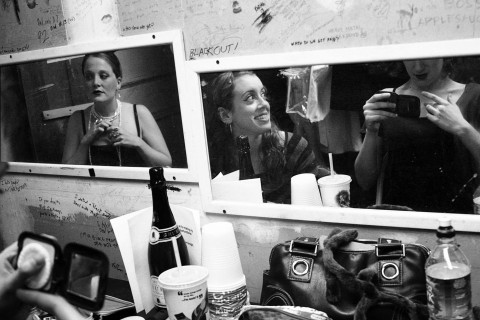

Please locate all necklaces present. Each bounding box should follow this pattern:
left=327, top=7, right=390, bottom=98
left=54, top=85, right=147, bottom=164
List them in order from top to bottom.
left=89, top=99, right=122, bottom=167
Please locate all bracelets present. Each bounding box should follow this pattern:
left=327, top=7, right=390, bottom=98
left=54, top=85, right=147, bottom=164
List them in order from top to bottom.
left=366, top=129, right=376, bottom=136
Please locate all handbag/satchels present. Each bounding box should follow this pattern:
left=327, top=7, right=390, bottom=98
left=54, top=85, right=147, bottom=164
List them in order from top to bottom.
left=260, top=234, right=431, bottom=320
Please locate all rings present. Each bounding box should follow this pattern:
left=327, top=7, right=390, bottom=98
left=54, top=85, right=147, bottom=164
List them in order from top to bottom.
left=111, top=130, right=119, bottom=137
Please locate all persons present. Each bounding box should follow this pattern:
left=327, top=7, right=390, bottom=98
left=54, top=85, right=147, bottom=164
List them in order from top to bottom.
left=61, top=50, right=172, bottom=166
left=354, top=57, right=480, bottom=215
left=207, top=70, right=318, bottom=206
left=0, top=234, right=87, bottom=320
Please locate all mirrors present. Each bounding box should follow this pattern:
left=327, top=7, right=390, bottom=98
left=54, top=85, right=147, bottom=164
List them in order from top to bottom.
left=0, top=31, right=197, bottom=185
left=186, top=38, right=480, bottom=233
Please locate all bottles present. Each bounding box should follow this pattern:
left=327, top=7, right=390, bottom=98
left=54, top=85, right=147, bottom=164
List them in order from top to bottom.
left=147, top=167, right=191, bottom=316
left=424, top=225, right=475, bottom=320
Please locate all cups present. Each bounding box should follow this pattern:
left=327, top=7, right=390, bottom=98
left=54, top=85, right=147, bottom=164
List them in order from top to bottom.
left=290, top=173, right=323, bottom=207
left=317, top=174, right=352, bottom=207
left=201, top=223, right=248, bottom=319
left=159, top=265, right=208, bottom=320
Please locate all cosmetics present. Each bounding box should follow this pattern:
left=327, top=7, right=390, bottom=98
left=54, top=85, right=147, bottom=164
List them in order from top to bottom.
left=17, top=231, right=109, bottom=311
